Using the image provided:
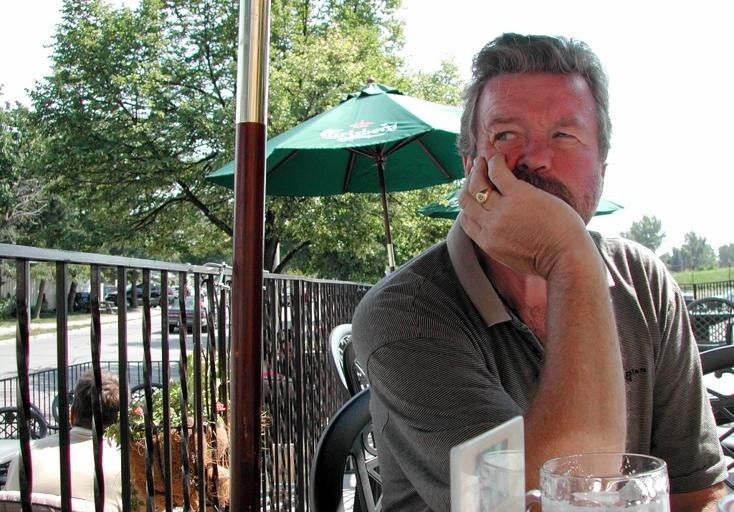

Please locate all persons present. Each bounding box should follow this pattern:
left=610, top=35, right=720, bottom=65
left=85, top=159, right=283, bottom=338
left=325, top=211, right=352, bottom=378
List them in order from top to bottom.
left=349, top=31, right=732, bottom=512
left=1, top=366, right=132, bottom=512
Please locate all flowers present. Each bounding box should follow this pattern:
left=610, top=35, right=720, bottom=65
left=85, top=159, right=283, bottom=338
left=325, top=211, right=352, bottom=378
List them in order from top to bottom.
left=106, top=344, right=292, bottom=508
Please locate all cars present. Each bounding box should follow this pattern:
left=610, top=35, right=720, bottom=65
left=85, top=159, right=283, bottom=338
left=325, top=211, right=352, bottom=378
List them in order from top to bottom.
left=75, top=282, right=207, bottom=333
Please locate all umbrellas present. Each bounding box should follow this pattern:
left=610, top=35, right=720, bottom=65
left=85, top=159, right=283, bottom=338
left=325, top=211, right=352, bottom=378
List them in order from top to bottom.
left=204, top=75, right=468, bottom=267
left=418, top=176, right=623, bottom=223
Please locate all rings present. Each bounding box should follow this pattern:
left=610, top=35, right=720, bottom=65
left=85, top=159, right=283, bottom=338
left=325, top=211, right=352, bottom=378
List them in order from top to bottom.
left=474, top=185, right=492, bottom=205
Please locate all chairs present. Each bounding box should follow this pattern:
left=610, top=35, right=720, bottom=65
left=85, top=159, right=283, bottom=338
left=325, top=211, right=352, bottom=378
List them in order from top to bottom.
left=687, top=296, right=734, bottom=346
left=326, top=321, right=377, bottom=512
left=698, top=340, right=734, bottom=446
left=297, top=383, right=398, bottom=511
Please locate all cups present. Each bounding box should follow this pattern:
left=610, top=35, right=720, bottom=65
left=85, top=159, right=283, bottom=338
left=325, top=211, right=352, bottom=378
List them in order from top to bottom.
left=540, top=450, right=673, bottom=512
left=478, top=452, right=523, bottom=511
